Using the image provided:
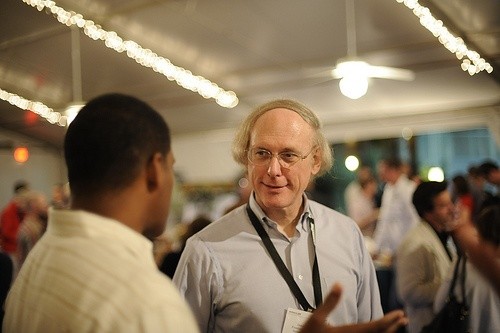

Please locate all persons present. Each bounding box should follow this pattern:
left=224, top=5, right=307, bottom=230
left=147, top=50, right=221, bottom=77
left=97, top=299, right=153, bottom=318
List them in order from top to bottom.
left=2, top=92, right=409, bottom=333
left=173, top=100, right=384, bottom=333
left=344, top=157, right=500, bottom=333
left=0, top=181, right=67, bottom=268
left=158, top=217, right=212, bottom=279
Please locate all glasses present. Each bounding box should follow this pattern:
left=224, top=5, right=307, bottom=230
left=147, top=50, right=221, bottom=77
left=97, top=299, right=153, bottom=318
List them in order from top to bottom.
left=247, top=145, right=317, bottom=169
